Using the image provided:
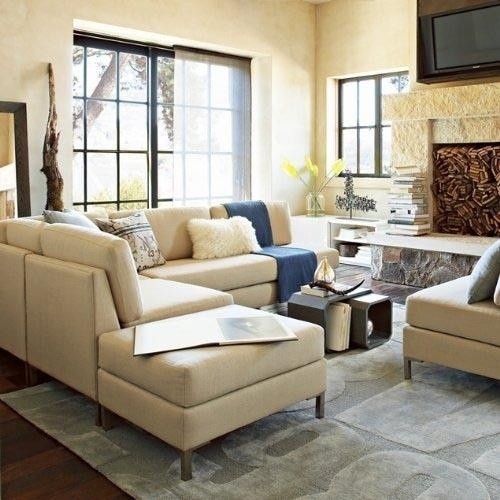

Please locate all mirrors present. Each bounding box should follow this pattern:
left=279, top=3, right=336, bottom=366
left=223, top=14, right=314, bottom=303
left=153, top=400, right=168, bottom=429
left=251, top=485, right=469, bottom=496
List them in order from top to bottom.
left=0, top=101, right=31, bottom=221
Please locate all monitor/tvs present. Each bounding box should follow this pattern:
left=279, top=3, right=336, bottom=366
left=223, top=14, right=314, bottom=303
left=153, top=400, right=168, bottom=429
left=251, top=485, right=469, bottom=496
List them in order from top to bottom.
left=417, top=1, right=500, bottom=75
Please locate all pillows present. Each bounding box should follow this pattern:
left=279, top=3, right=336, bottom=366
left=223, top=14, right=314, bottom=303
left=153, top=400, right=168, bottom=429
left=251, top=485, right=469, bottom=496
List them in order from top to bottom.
left=6, top=210, right=262, bottom=321
left=467, top=239, right=500, bottom=306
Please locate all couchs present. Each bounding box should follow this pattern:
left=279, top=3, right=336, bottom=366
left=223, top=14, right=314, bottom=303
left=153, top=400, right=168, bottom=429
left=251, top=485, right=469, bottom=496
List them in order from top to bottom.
left=403, top=274, right=500, bottom=380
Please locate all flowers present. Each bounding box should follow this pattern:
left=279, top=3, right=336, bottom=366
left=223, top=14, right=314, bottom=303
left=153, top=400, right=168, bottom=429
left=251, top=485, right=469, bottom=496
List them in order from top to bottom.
left=282, top=154, right=344, bottom=215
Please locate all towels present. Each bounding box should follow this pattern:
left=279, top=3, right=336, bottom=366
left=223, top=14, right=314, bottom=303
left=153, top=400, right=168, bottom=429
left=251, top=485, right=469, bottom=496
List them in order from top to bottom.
left=220, top=200, right=317, bottom=304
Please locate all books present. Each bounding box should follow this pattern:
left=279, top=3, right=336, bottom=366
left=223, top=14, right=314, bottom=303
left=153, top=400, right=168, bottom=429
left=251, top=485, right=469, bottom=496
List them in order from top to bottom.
left=385, top=166, right=431, bottom=236
left=338, top=227, right=368, bottom=240
left=354, top=245, right=372, bottom=265
left=300, top=284, right=336, bottom=297
left=326, top=301, right=352, bottom=351
left=131, top=314, right=299, bottom=357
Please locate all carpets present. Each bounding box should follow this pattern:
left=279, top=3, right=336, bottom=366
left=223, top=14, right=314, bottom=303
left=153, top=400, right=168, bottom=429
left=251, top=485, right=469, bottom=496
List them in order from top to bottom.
left=1, top=303, right=500, bottom=500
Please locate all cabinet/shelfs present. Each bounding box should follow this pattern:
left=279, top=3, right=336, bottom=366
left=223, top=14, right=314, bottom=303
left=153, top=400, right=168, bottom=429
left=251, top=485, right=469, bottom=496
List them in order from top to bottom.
left=291, top=215, right=388, bottom=268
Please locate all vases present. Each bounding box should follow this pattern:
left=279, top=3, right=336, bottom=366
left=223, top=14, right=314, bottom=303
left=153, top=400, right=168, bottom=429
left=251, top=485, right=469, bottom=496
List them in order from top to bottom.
left=308, top=195, right=325, bottom=217
